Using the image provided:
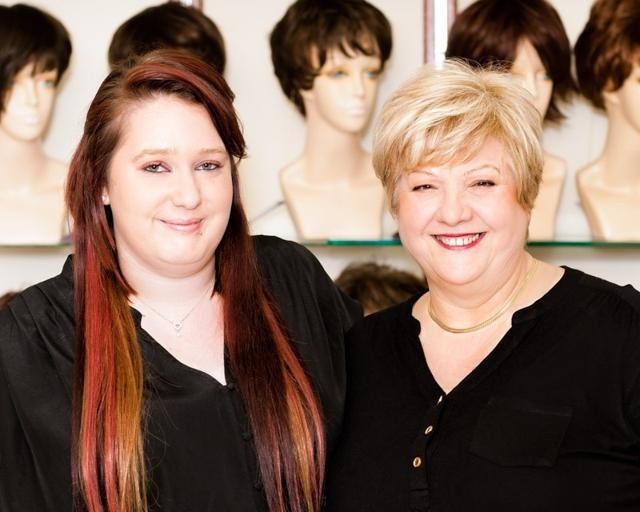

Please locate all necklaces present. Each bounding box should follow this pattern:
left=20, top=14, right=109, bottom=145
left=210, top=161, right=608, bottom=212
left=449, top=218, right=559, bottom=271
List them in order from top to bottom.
left=425, top=256, right=542, bottom=334
left=130, top=281, right=217, bottom=334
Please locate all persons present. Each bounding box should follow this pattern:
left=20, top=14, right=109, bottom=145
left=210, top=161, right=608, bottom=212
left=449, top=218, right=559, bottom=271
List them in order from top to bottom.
left=444, top=0, right=578, bottom=240
left=269, top=1, right=392, bottom=242
left=572, top=1, right=640, bottom=243
left=0, top=3, right=75, bottom=245
left=107, top=4, right=227, bottom=81
left=0, top=51, right=365, bottom=512
left=325, top=59, right=640, bottom=511
left=332, top=257, right=427, bottom=321
